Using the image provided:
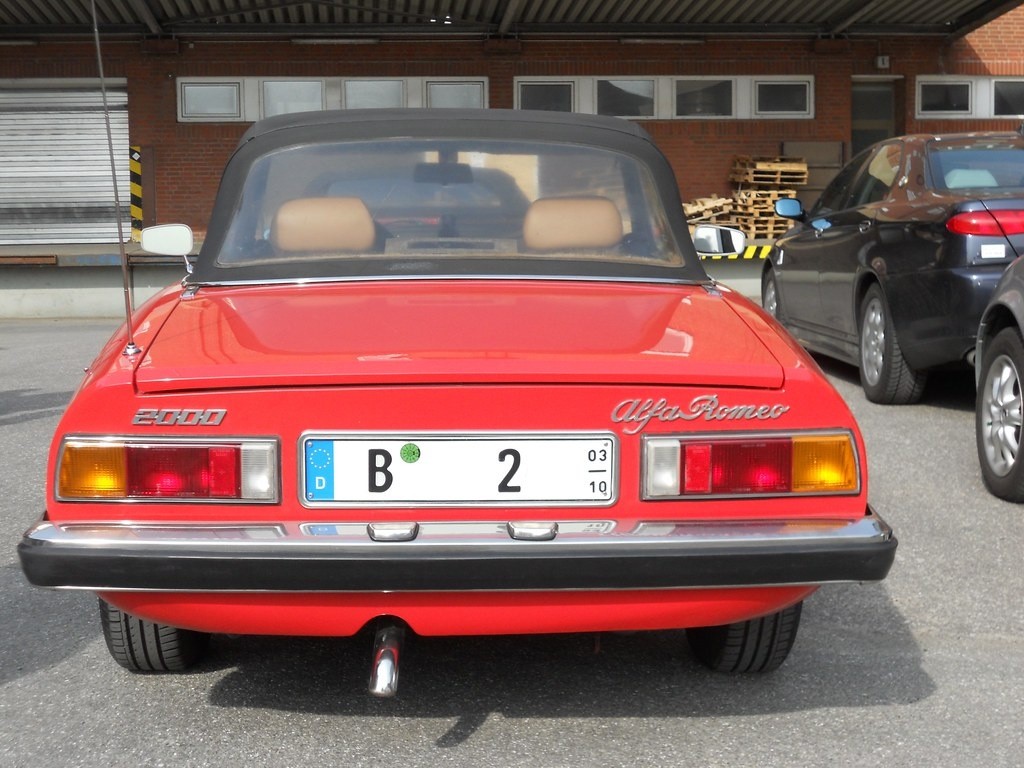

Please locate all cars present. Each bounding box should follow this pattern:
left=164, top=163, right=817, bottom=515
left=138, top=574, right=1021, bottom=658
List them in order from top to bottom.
left=17, top=108, right=898, bottom=676
left=761, top=124, right=1024, bottom=405
left=975, top=255, right=1024, bottom=503
